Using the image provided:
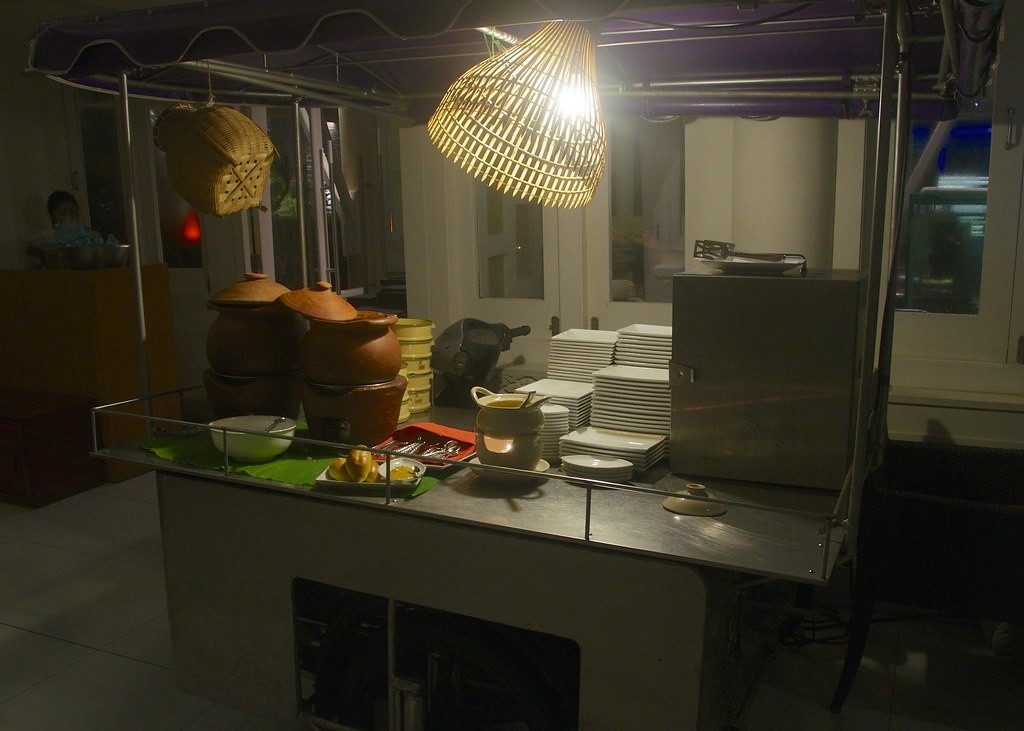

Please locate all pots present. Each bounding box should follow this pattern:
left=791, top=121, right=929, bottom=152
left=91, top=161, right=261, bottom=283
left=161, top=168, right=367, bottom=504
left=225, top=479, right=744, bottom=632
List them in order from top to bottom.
left=200, top=271, right=409, bottom=448
left=471, top=386, right=551, bottom=439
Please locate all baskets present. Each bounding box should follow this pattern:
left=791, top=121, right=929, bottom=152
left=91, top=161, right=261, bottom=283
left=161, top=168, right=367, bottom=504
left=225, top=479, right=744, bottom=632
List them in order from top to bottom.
left=151, top=102, right=281, bottom=219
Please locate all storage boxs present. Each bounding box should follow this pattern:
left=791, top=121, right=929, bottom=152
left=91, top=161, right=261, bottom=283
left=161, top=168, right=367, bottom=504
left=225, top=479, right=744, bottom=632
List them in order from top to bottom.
left=0, top=386, right=107, bottom=510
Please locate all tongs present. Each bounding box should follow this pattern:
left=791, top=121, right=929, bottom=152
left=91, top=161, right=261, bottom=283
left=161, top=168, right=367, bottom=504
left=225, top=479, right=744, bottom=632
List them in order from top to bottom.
left=695, top=240, right=808, bottom=262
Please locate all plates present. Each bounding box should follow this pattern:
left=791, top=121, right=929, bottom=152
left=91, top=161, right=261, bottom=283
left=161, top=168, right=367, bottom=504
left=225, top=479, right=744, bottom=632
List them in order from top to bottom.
left=701, top=257, right=805, bottom=275
left=515, top=323, right=673, bottom=485
left=469, top=458, right=551, bottom=484
left=315, top=465, right=423, bottom=490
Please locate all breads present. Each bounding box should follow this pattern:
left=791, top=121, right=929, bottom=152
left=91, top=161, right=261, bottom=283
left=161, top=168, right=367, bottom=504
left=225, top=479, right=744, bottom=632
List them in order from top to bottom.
left=328, top=444, right=381, bottom=483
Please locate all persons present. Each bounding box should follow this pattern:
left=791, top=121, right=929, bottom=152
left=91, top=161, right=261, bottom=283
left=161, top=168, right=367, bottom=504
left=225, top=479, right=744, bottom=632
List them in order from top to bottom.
left=36, top=190, right=103, bottom=247
left=926, top=212, right=984, bottom=286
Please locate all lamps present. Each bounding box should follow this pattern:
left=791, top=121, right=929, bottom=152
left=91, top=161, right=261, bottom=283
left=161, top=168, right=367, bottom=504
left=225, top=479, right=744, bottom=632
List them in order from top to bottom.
left=425, top=21, right=610, bottom=209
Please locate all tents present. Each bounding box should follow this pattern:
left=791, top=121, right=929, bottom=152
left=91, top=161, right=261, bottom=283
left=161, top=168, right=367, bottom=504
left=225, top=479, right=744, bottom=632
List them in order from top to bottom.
left=19, top=0, right=1006, bottom=578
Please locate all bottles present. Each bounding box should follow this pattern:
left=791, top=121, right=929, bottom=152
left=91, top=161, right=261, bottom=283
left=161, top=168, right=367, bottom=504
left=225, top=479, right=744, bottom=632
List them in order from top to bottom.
left=50, top=223, right=121, bottom=245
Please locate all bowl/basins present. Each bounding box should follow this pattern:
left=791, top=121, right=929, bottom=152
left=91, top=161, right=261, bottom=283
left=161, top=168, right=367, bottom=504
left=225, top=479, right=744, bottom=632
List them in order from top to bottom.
left=73, top=244, right=130, bottom=269
left=38, top=247, right=80, bottom=270
left=394, top=318, right=436, bottom=424
left=209, top=414, right=298, bottom=463
left=378, top=458, right=426, bottom=482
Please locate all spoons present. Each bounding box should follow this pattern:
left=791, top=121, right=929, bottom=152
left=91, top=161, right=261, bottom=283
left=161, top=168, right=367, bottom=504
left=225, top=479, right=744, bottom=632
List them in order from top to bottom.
left=419, top=437, right=461, bottom=463
